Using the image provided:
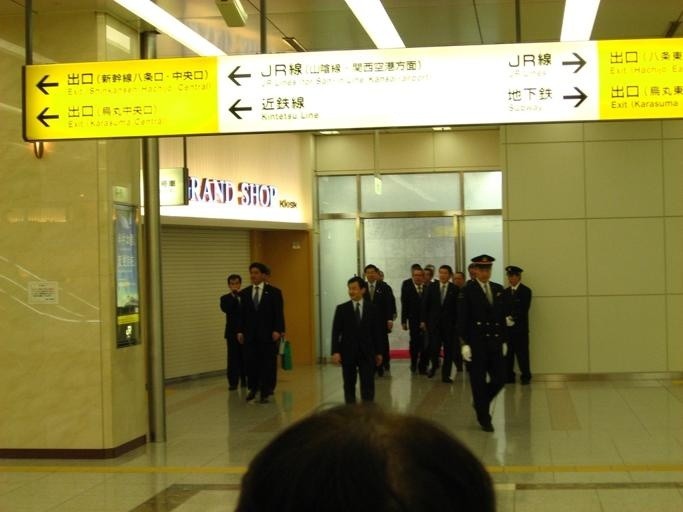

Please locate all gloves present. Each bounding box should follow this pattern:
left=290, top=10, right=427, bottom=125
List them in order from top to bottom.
left=505, top=315, right=516, bottom=326
left=502, top=342, right=508, bottom=357
left=460, top=344, right=473, bottom=362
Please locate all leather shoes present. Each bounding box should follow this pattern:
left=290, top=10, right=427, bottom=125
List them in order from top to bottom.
left=409, top=360, right=534, bottom=384
left=228, top=383, right=272, bottom=403
left=475, top=415, right=495, bottom=433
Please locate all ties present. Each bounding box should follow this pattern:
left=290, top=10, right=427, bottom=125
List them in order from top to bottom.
left=369, top=284, right=375, bottom=301
left=483, top=284, right=493, bottom=304
left=440, top=285, right=446, bottom=305
left=253, top=286, right=259, bottom=308
left=418, top=286, right=422, bottom=297
left=512, top=289, right=515, bottom=296
left=234, top=294, right=239, bottom=305
left=355, top=302, right=361, bottom=325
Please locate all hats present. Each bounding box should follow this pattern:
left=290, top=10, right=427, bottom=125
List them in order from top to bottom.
left=470, top=254, right=495, bottom=265
left=505, top=265, right=523, bottom=274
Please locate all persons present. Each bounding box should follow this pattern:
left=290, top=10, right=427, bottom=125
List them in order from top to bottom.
left=449, top=255, right=511, bottom=430
left=220, top=262, right=286, bottom=400
left=331, top=264, right=397, bottom=403
left=401, top=264, right=465, bottom=382
left=504, top=265, right=532, bottom=385
left=235, top=401, right=497, bottom=512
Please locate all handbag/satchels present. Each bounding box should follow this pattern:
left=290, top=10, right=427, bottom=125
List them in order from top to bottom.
left=278, top=334, right=294, bottom=372
